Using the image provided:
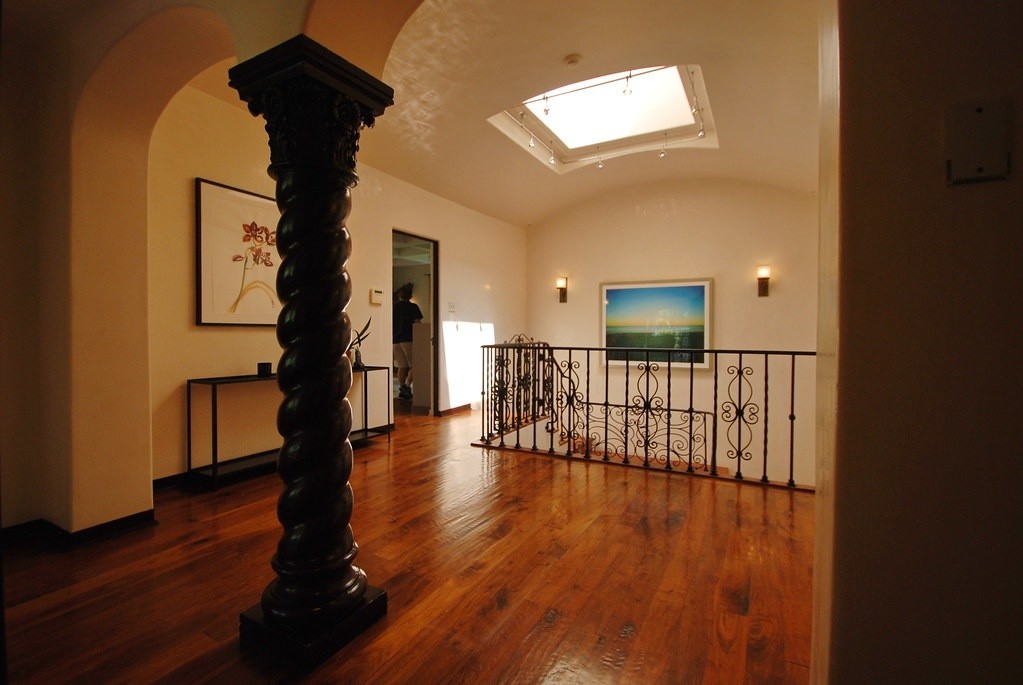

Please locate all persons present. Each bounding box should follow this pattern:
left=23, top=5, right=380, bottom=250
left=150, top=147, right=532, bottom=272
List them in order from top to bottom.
left=393, top=287, right=423, bottom=399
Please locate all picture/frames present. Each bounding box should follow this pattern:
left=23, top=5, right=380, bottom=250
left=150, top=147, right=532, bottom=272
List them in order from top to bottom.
left=194, top=177, right=281, bottom=325
left=598, top=277, right=714, bottom=369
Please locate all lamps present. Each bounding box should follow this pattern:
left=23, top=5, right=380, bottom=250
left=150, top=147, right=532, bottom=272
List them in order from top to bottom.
left=756, top=265, right=772, bottom=298
left=555, top=276, right=567, bottom=302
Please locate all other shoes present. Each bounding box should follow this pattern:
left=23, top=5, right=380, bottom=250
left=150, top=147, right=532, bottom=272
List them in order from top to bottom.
left=399, top=384, right=413, bottom=400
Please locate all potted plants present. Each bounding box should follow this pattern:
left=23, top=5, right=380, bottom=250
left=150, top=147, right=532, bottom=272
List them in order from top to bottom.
left=346, top=317, right=374, bottom=364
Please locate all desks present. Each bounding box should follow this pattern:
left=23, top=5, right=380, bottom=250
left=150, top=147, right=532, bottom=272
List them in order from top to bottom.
left=181, top=365, right=394, bottom=492
